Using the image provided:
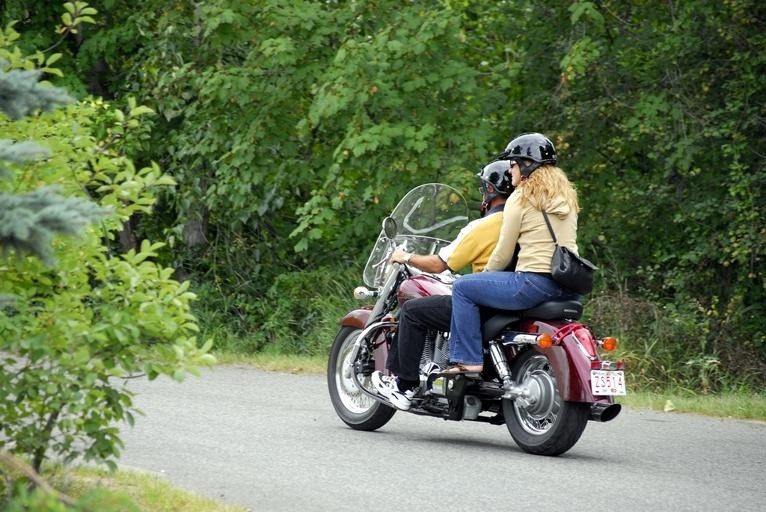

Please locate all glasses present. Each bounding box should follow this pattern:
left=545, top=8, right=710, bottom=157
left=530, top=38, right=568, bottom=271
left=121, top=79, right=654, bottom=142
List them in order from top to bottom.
left=510, top=160, right=518, bottom=168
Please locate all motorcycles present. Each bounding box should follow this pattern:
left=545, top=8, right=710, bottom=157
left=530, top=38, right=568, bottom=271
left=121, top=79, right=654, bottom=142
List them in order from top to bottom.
left=325, top=183, right=628, bottom=454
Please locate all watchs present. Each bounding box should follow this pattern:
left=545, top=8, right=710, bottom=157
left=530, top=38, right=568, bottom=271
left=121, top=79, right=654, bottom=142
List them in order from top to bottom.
left=403, top=252, right=413, bottom=263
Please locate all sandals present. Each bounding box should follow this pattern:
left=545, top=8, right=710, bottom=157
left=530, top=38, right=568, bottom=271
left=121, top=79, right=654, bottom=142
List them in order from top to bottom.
left=439, top=364, right=483, bottom=375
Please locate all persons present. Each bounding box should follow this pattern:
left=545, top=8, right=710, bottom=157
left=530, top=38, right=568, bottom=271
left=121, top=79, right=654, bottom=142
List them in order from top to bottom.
left=441, top=132, right=580, bottom=376
left=370, top=160, right=519, bottom=411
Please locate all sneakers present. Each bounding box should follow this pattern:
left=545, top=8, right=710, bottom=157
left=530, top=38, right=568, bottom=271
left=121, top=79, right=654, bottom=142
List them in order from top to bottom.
left=371, top=369, right=416, bottom=411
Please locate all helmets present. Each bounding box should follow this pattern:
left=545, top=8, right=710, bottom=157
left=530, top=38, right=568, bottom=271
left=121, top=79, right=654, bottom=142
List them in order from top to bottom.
left=496, top=131, right=558, bottom=166
left=476, top=159, right=515, bottom=197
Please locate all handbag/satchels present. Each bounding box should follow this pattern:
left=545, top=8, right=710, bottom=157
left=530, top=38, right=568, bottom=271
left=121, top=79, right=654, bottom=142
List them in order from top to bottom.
left=549, top=244, right=599, bottom=296
